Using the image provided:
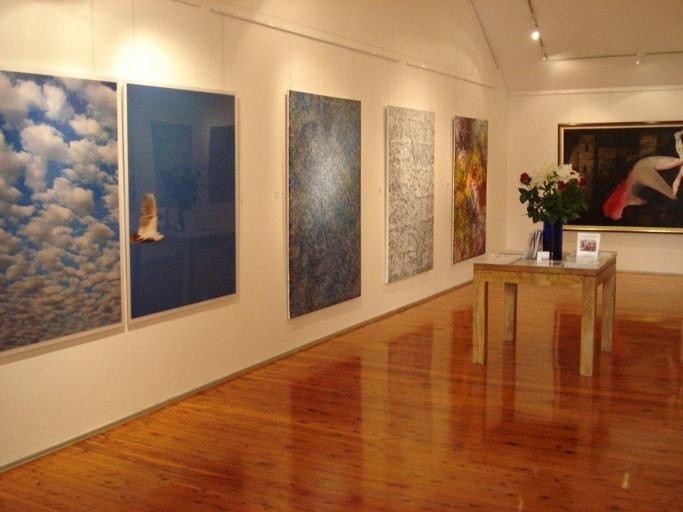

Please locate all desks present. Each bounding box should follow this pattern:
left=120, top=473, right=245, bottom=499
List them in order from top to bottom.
left=473, top=252, right=617, bottom=377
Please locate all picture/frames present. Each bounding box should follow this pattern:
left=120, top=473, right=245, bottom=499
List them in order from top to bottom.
left=557, top=121, right=683, bottom=234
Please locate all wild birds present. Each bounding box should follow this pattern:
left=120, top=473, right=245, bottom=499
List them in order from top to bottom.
left=129, top=192, right=164, bottom=245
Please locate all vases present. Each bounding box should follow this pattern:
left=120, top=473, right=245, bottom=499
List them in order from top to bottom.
left=544, top=220, right=563, bottom=261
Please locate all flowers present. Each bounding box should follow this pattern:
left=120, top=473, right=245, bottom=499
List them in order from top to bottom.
left=517, top=162, right=589, bottom=224
